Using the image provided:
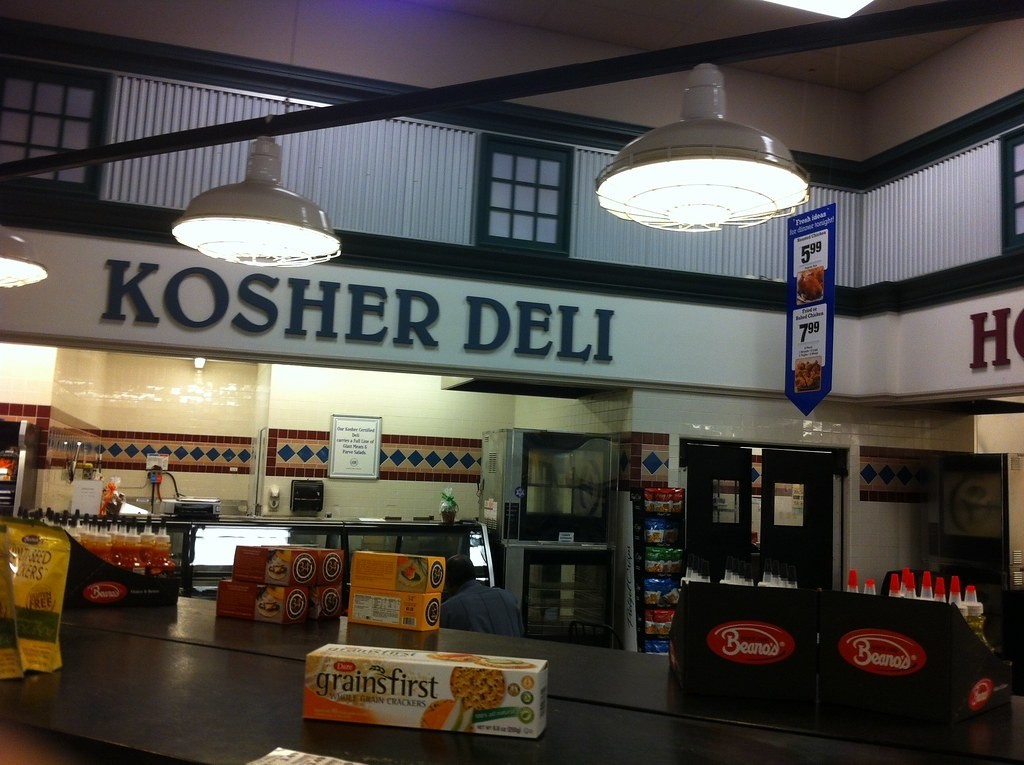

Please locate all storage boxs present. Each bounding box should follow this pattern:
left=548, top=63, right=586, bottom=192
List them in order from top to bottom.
left=64, top=529, right=180, bottom=613
left=215, top=545, right=445, bottom=631
left=666, top=580, right=820, bottom=704
left=818, top=590, right=1012, bottom=725
left=303, top=644, right=549, bottom=739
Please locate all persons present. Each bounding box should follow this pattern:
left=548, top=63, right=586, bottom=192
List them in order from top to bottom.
left=441, top=553, right=522, bottom=640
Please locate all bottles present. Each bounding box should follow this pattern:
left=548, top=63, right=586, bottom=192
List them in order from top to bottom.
left=17, top=507, right=172, bottom=575
left=684, top=553, right=711, bottom=582
left=724, top=559, right=754, bottom=585
left=763, top=560, right=797, bottom=588
left=901, top=567, right=910, bottom=597
left=846, top=569, right=858, bottom=592
left=921, top=572, right=933, bottom=599
left=904, top=573, right=916, bottom=599
left=889, top=574, right=901, bottom=597
left=949, top=576, right=967, bottom=620
left=935, top=578, right=946, bottom=602
left=864, top=579, right=876, bottom=595
left=964, top=586, right=994, bottom=653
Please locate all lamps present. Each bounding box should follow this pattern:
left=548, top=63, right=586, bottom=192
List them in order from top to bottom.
left=594, top=61, right=812, bottom=236
left=169, top=133, right=342, bottom=269
left=0, top=229, right=50, bottom=292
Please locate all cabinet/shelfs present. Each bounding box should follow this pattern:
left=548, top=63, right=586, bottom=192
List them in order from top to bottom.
left=614, top=487, right=687, bottom=652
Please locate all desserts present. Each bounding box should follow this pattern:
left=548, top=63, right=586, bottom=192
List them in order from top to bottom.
left=399, top=562, right=423, bottom=587
left=257, top=563, right=291, bottom=616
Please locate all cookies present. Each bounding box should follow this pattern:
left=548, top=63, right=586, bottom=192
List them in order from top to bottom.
left=427, top=654, right=533, bottom=711
